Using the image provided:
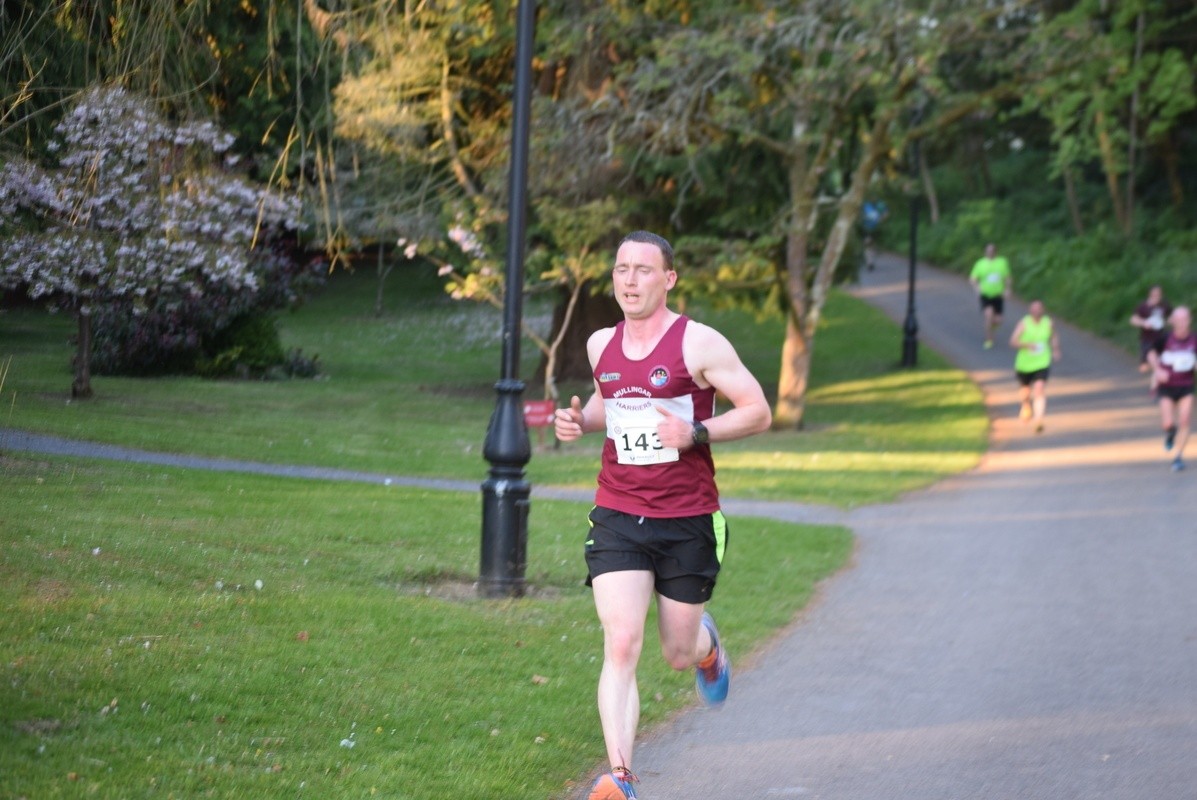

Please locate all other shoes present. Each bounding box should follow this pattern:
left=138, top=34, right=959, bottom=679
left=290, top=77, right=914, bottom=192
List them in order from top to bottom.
left=983, top=339, right=992, bottom=350
left=1020, top=407, right=1032, bottom=421
left=1035, top=423, right=1043, bottom=434
left=1165, top=426, right=1177, bottom=448
left=1171, top=459, right=1184, bottom=471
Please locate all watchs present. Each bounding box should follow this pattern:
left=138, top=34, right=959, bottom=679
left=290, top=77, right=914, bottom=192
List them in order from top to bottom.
left=692, top=422, right=709, bottom=447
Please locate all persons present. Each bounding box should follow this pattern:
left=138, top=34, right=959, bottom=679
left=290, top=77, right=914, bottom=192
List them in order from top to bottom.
left=863, top=200, right=887, bottom=270
left=554, top=231, right=773, bottom=799
left=970, top=241, right=1014, bottom=351
left=1129, top=285, right=1174, bottom=383
left=1007, top=299, right=1060, bottom=436
left=1147, top=307, right=1197, bottom=471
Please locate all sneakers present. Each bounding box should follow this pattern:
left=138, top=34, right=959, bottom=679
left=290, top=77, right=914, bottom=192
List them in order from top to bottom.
left=694, top=609, right=728, bottom=702
left=586, top=751, right=640, bottom=800
